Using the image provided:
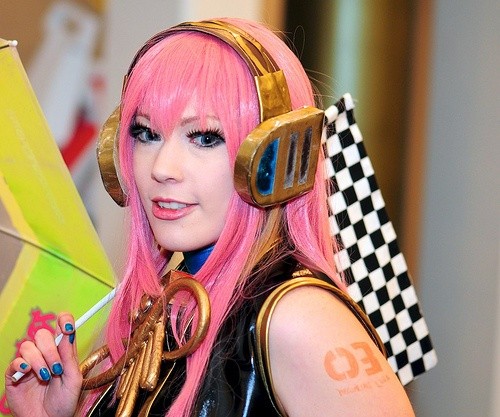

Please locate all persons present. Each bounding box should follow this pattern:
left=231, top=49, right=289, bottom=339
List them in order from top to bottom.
left=3, top=15, right=419, bottom=417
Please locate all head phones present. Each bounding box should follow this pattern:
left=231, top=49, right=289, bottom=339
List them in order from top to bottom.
left=96, top=19, right=326, bottom=207
